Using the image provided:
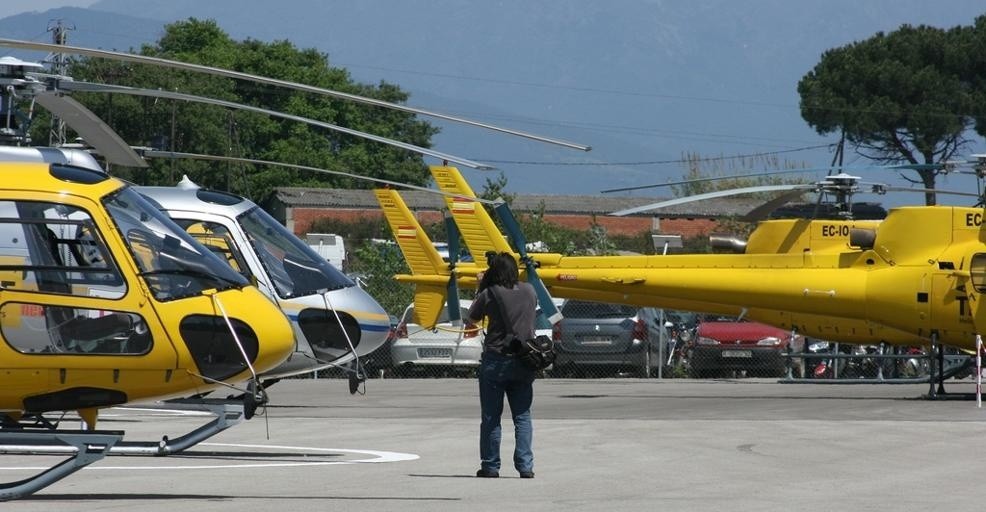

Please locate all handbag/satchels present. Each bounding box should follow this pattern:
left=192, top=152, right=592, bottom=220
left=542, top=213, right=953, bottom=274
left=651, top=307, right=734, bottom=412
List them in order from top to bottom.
left=511, top=335, right=557, bottom=370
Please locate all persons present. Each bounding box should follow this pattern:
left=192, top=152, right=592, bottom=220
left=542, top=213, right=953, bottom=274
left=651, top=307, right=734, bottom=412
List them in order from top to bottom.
left=468, top=252, right=538, bottom=478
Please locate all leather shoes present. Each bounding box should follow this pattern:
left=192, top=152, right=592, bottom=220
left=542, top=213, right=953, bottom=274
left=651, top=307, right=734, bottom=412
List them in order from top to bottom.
left=477, top=470, right=499, bottom=478
left=520, top=472, right=535, bottom=478
